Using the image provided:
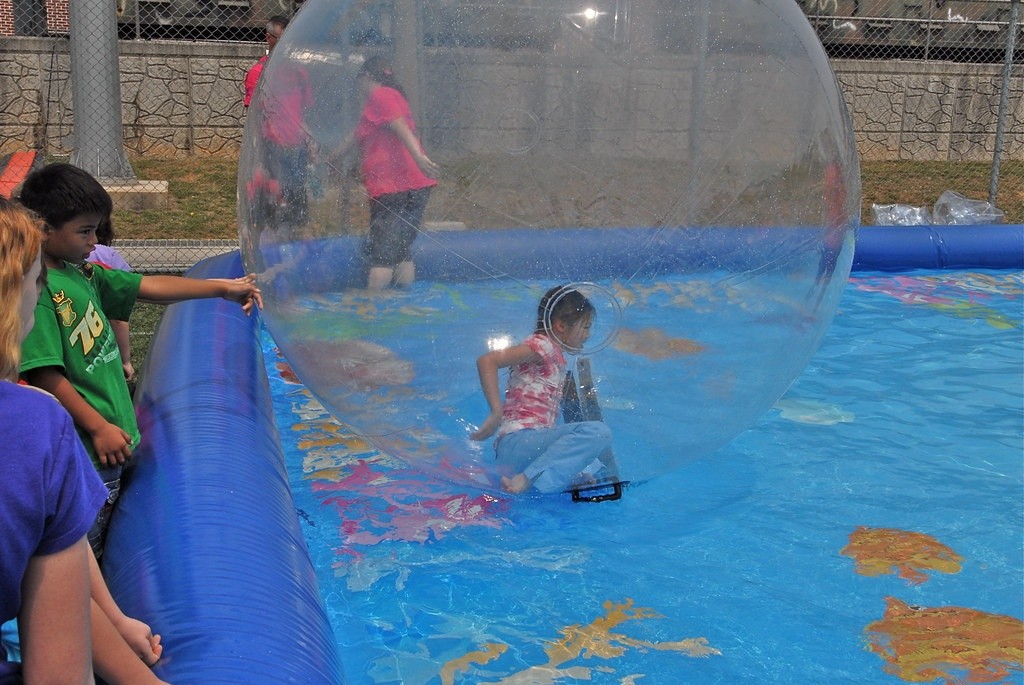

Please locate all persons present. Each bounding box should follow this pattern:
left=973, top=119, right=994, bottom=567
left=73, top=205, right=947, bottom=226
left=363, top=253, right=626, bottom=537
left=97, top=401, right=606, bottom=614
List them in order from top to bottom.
left=240, top=14, right=314, bottom=283
left=323, top=55, right=442, bottom=295
left=0, top=150, right=270, bottom=685
left=470, top=286, right=619, bottom=494
